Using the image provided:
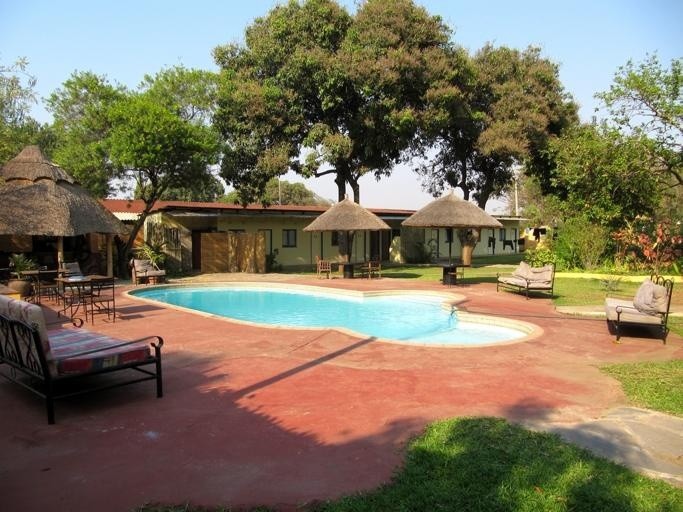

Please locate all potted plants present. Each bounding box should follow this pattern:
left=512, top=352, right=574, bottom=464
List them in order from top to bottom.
left=8, top=253, right=36, bottom=296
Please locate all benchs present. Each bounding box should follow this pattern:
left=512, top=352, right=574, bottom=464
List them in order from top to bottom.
left=496, top=259, right=557, bottom=299
left=606, top=270, right=674, bottom=344
left=0, top=293, right=164, bottom=426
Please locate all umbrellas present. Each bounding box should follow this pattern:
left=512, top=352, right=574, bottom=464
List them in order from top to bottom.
left=0, top=144, right=130, bottom=274
left=398, top=190, right=507, bottom=263
left=303, top=194, right=392, bottom=264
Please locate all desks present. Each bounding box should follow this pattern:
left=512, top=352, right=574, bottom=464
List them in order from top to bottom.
left=331, top=261, right=367, bottom=278
left=430, top=264, right=470, bottom=285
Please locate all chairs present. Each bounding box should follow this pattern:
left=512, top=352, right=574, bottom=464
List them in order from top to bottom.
left=360, top=254, right=381, bottom=279
left=314, top=256, right=332, bottom=280
left=128, top=257, right=168, bottom=285
left=447, top=257, right=465, bottom=289
left=18, top=265, right=118, bottom=324
